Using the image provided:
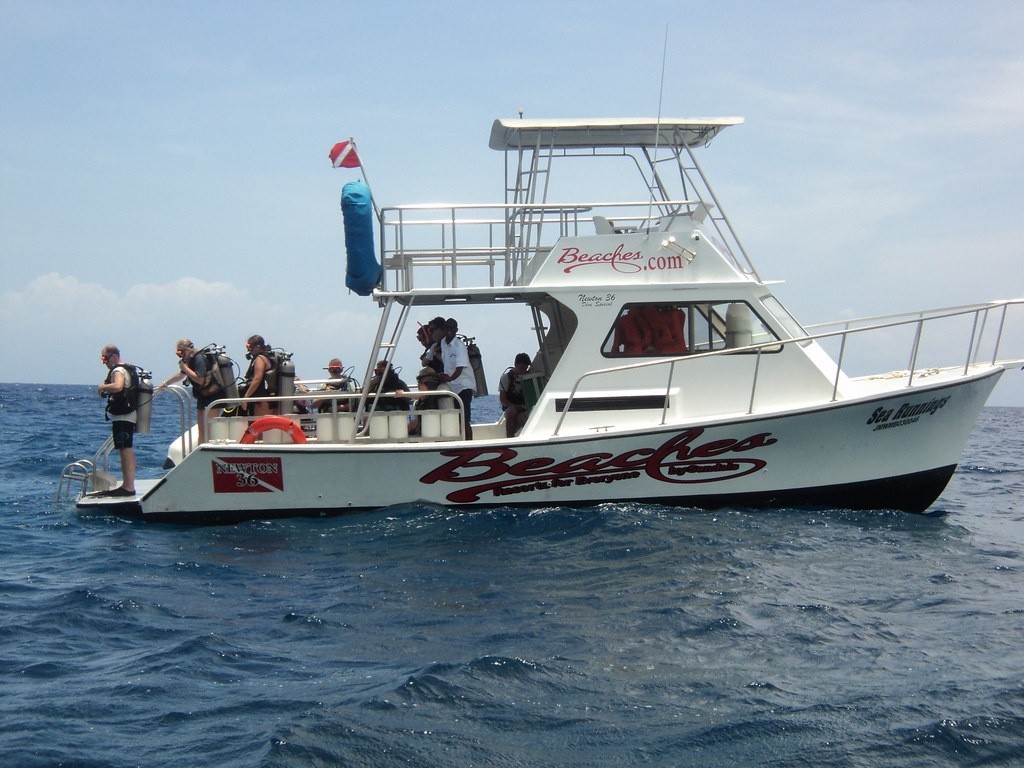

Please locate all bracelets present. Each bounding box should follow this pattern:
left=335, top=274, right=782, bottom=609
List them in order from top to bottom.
left=163, top=381, right=167, bottom=386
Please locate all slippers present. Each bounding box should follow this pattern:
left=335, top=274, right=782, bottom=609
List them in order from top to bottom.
left=107, top=487, right=136, bottom=495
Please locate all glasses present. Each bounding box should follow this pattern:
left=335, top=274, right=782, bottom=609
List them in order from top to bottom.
left=427, top=327, right=434, bottom=334
left=417, top=335, right=424, bottom=341
left=246, top=344, right=253, bottom=351
left=176, top=350, right=184, bottom=358
left=101, top=357, right=108, bottom=363
left=328, top=366, right=342, bottom=373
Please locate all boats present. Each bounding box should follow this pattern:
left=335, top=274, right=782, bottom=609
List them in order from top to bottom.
left=55, top=19, right=1024, bottom=526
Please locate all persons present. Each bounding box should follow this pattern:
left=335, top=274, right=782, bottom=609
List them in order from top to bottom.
left=409, top=316, right=476, bottom=440
left=242, top=335, right=277, bottom=415
left=159, top=338, right=220, bottom=445
left=98, top=345, right=136, bottom=496
left=500, top=353, right=532, bottom=437
left=312, top=358, right=357, bottom=413
left=363, top=360, right=409, bottom=436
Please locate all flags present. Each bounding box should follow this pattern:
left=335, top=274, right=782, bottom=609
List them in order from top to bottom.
left=329, top=141, right=361, bottom=168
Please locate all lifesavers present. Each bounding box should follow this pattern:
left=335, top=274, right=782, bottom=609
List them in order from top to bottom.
left=239, top=415, right=307, bottom=444
